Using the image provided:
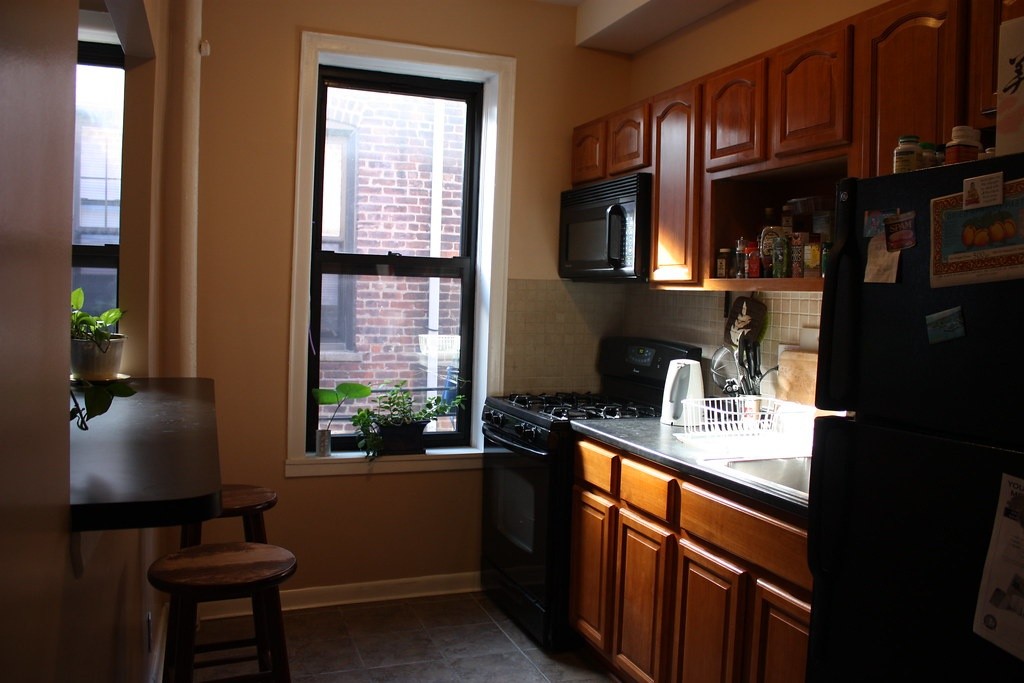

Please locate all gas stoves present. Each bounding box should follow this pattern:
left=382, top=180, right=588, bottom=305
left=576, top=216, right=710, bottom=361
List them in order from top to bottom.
left=484, top=335, right=702, bottom=429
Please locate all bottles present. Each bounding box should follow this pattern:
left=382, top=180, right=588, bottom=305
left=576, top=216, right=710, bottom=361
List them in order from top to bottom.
left=893, top=126, right=981, bottom=173
left=717, top=204, right=796, bottom=278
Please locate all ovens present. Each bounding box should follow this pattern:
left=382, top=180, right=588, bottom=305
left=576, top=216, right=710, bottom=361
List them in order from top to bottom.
left=480, top=404, right=573, bottom=653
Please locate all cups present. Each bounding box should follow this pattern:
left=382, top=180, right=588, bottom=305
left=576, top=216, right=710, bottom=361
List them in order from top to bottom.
left=738, top=392, right=762, bottom=429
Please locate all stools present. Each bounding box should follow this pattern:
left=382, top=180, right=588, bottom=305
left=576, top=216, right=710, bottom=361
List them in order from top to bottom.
left=178, top=482, right=278, bottom=683
left=147, top=541, right=298, bottom=683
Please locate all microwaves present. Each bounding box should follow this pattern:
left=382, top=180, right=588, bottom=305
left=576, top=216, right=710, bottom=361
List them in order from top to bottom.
left=557, top=171, right=654, bottom=283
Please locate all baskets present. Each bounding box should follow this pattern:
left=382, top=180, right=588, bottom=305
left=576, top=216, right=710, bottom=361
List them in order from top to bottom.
left=680, top=393, right=815, bottom=459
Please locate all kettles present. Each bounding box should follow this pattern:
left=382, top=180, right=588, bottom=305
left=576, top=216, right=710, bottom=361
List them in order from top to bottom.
left=659, top=359, right=705, bottom=426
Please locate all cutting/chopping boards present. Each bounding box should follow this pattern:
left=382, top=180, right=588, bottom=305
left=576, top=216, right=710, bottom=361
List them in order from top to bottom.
left=776, top=351, right=818, bottom=406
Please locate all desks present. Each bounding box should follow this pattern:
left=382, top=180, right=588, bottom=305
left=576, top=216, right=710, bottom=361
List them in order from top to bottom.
left=69, top=377, right=222, bottom=533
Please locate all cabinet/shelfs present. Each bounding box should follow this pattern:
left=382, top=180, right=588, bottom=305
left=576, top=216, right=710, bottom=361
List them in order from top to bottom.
left=847, top=0, right=1024, bottom=178
left=703, top=15, right=852, bottom=182
left=648, top=78, right=705, bottom=292
left=571, top=96, right=654, bottom=188
left=567, top=434, right=680, bottom=683
left=667, top=474, right=813, bottom=683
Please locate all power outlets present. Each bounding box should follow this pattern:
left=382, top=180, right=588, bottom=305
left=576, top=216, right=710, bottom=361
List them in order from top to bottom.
left=776, top=344, right=801, bottom=376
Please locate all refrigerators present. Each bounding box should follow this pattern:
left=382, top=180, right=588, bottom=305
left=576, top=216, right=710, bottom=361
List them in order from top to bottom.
left=801, top=152, right=1024, bottom=683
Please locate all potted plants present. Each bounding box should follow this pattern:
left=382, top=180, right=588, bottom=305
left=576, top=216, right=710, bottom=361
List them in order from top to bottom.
left=71, top=287, right=128, bottom=380
left=349, top=377, right=467, bottom=464
left=311, top=381, right=372, bottom=458
